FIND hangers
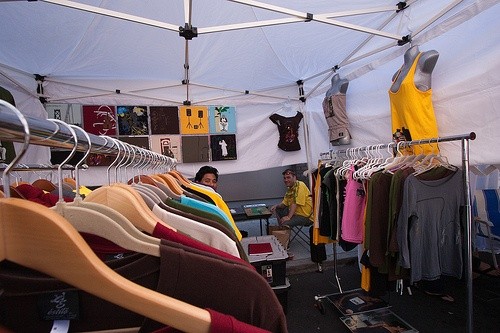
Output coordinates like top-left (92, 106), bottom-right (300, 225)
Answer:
top-left (0, 97), bottom-right (211, 332)
top-left (317, 137), bottom-right (458, 180)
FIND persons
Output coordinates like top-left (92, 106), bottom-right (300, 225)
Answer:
top-left (389, 45), bottom-right (440, 155)
top-left (270, 168), bottom-right (314, 251)
top-left (269, 104), bottom-right (304, 153)
top-left (322, 73), bottom-right (352, 146)
top-left (194, 166), bottom-right (218, 190)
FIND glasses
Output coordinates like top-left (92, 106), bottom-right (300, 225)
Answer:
top-left (282, 172), bottom-right (295, 175)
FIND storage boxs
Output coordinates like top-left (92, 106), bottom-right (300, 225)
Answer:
top-left (271, 277), bottom-right (291, 314)
top-left (241, 235), bottom-right (290, 287)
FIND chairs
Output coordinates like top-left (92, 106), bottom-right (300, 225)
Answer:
top-left (471, 189), bottom-right (500, 270)
top-left (278, 208), bottom-right (313, 251)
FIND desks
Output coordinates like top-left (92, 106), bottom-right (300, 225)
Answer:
top-left (229, 207), bottom-right (272, 236)
